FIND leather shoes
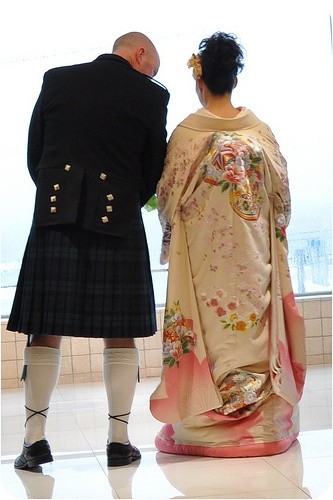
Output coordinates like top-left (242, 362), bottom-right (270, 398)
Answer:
top-left (14, 437), bottom-right (53, 470)
top-left (107, 438), bottom-right (142, 466)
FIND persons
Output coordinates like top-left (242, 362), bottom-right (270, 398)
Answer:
top-left (5, 31), bottom-right (169, 468)
top-left (148, 31), bottom-right (306, 457)
